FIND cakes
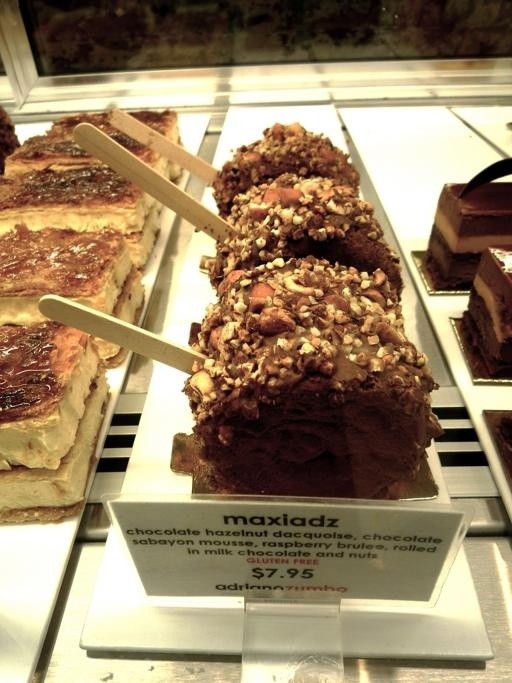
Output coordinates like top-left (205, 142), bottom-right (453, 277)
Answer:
top-left (208, 170), bottom-right (404, 301)
top-left (461, 245), bottom-right (512, 378)
top-left (0, 224), bottom-right (145, 367)
top-left (212, 122), bottom-right (361, 222)
top-left (47, 111), bottom-right (185, 186)
top-left (4, 135), bottom-right (172, 178)
top-left (185, 254), bottom-right (443, 500)
top-left (425, 182), bottom-right (512, 291)
top-left (0, 323), bottom-right (109, 524)
top-left (0, 167), bottom-right (164, 265)
